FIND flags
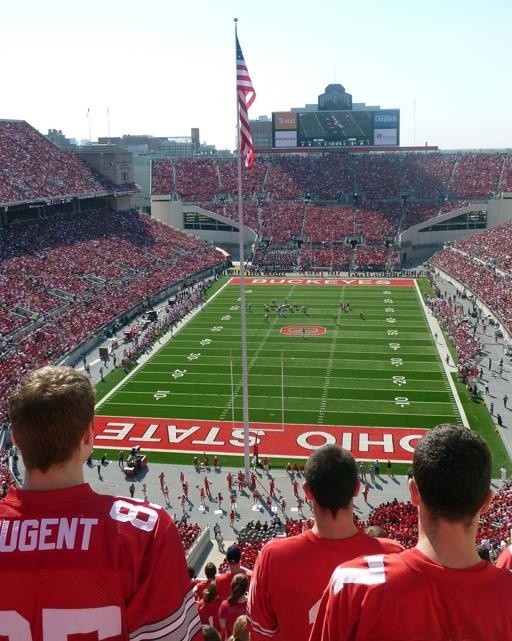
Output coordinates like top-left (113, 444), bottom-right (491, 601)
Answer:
top-left (235, 33), bottom-right (259, 170)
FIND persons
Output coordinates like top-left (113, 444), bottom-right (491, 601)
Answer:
top-left (1, 121), bottom-right (512, 640)
top-left (310, 425), bottom-right (512, 641)
top-left (1, 364), bottom-right (202, 641)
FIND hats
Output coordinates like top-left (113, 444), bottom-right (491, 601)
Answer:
top-left (226, 544), bottom-right (241, 563)
